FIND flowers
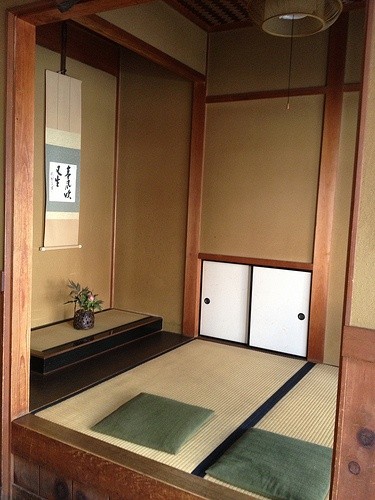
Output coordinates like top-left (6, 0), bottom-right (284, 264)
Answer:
top-left (64, 280), bottom-right (103, 314)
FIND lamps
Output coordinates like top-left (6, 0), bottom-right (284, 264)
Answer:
top-left (247, 0), bottom-right (342, 36)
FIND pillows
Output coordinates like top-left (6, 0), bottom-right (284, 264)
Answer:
top-left (90, 392), bottom-right (215, 455)
top-left (205, 428), bottom-right (333, 500)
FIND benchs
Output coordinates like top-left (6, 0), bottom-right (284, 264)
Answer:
top-left (29, 308), bottom-right (162, 375)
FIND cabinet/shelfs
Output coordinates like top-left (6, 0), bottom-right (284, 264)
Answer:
top-left (199, 258), bottom-right (311, 358)
